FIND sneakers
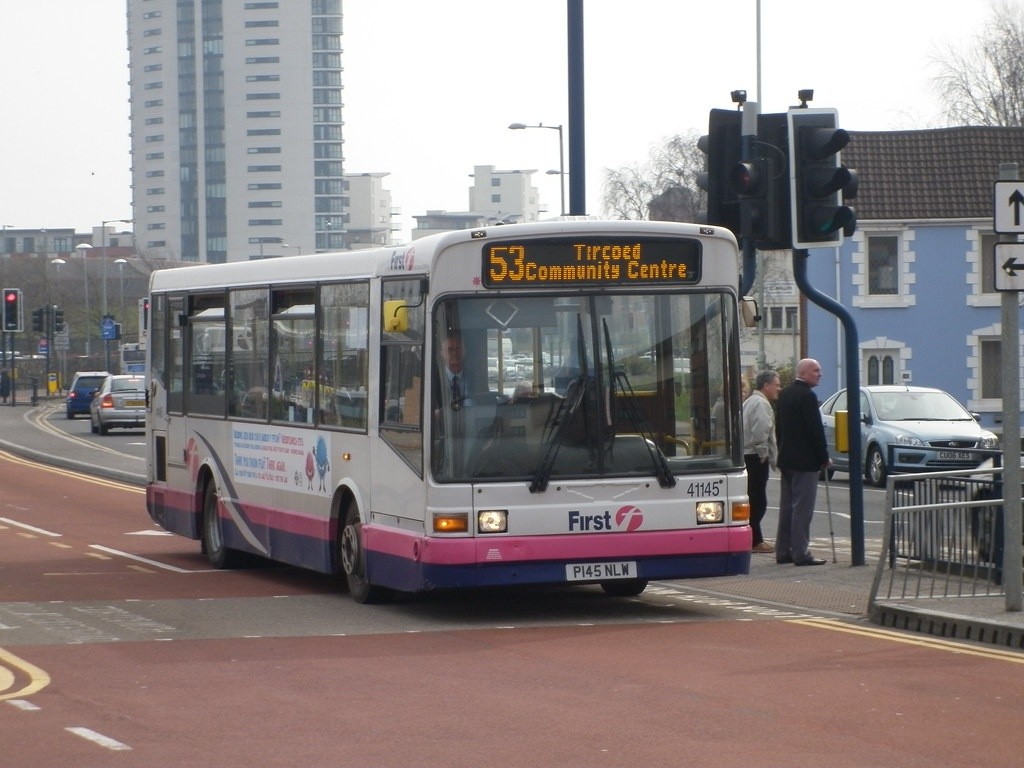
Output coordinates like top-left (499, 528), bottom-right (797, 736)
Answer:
top-left (752, 542), bottom-right (776, 552)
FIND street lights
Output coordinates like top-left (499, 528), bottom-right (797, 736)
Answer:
top-left (75, 219), bottom-right (131, 370)
top-left (509, 122), bottom-right (564, 216)
top-left (50, 259), bottom-right (66, 288)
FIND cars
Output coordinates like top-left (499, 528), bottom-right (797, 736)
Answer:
top-left (965, 446), bottom-right (1024, 565)
top-left (0, 350), bottom-right (47, 360)
top-left (89, 374), bottom-right (146, 435)
top-left (601, 347), bottom-right (691, 380)
top-left (486, 350), bottom-right (564, 382)
top-left (819, 384), bottom-right (999, 488)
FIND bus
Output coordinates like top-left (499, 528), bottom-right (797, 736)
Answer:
top-left (190, 325), bottom-right (280, 403)
top-left (120, 343), bottom-right (145, 374)
top-left (146, 217), bottom-right (762, 604)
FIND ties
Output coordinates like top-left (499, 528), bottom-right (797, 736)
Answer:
top-left (452, 376), bottom-right (460, 401)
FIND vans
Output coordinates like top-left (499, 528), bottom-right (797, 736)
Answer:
top-left (487, 338), bottom-right (512, 356)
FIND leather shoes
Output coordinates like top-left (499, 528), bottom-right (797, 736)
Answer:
top-left (777, 557), bottom-right (793, 564)
top-left (795, 556), bottom-right (826, 566)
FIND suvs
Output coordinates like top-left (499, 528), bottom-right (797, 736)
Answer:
top-left (66, 371), bottom-right (109, 419)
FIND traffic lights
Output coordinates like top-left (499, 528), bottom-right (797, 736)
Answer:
top-left (787, 108), bottom-right (853, 249)
top-left (698, 108), bottom-right (742, 233)
top-left (53, 305), bottom-right (64, 333)
top-left (33, 308), bottom-right (44, 331)
top-left (3, 288), bottom-right (20, 332)
top-left (735, 156), bottom-right (774, 239)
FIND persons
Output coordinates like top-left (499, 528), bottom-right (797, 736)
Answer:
top-left (743, 370), bottom-right (781, 552)
top-left (711, 377), bottom-right (750, 453)
top-left (513, 380), bottom-right (538, 398)
top-left (0, 367), bottom-right (10, 403)
top-left (775, 358), bottom-right (833, 567)
top-left (434, 336), bottom-right (513, 416)
